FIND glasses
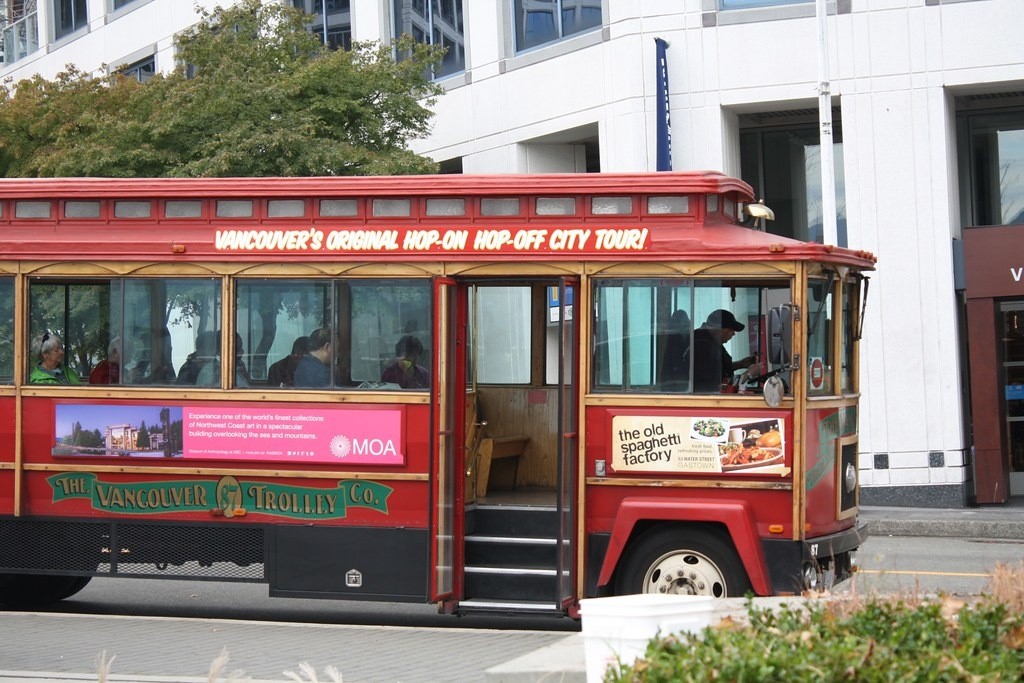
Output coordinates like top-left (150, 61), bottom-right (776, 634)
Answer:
top-left (39, 333), bottom-right (49, 354)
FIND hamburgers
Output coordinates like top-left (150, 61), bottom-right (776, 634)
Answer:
top-left (756, 431), bottom-right (781, 449)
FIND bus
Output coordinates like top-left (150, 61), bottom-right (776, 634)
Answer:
top-left (0, 171), bottom-right (880, 623)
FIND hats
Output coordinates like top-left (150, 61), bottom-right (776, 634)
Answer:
top-left (707, 310), bottom-right (745, 332)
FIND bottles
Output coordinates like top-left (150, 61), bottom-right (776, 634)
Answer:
top-left (769, 423), bottom-right (778, 431)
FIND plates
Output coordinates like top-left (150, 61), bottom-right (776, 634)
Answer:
top-left (719, 447), bottom-right (783, 471)
top-left (691, 418), bottom-right (729, 443)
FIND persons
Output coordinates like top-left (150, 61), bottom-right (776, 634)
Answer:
top-left (29, 332), bottom-right (81, 385)
top-left (266, 336), bottom-right (310, 387)
top-left (293, 328), bottom-right (346, 388)
top-left (175, 330), bottom-right (216, 385)
top-left (684, 308), bottom-right (765, 394)
top-left (197, 329), bottom-right (251, 388)
top-left (382, 335), bottom-right (433, 390)
top-left (89, 336), bottom-right (133, 384)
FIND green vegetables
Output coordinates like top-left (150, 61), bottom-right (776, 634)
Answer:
top-left (695, 420), bottom-right (725, 437)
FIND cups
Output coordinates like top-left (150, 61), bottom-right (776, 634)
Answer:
top-left (729, 428), bottom-right (747, 442)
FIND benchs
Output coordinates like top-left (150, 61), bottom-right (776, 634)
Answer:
top-left (465, 388), bottom-right (529, 498)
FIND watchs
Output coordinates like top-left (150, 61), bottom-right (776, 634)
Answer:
top-left (745, 371), bottom-right (753, 380)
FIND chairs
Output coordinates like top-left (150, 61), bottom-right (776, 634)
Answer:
top-left (662, 310), bottom-right (695, 394)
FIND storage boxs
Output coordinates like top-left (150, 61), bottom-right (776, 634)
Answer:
top-left (577, 595), bottom-right (710, 683)
